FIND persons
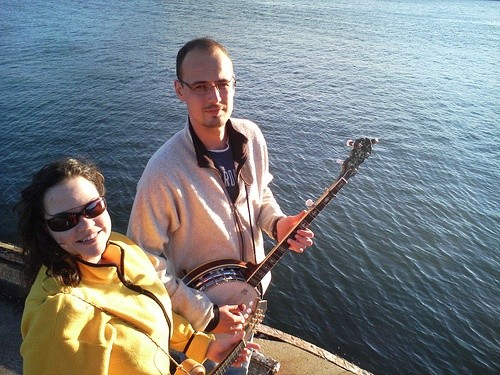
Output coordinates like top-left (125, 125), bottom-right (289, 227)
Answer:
top-left (21, 156), bottom-right (261, 375)
top-left (126, 37), bottom-right (314, 374)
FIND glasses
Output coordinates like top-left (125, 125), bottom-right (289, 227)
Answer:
top-left (45, 196), bottom-right (107, 232)
top-left (181, 76), bottom-right (237, 94)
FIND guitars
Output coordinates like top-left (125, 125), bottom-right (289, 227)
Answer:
top-left (168, 296), bottom-right (270, 375)
